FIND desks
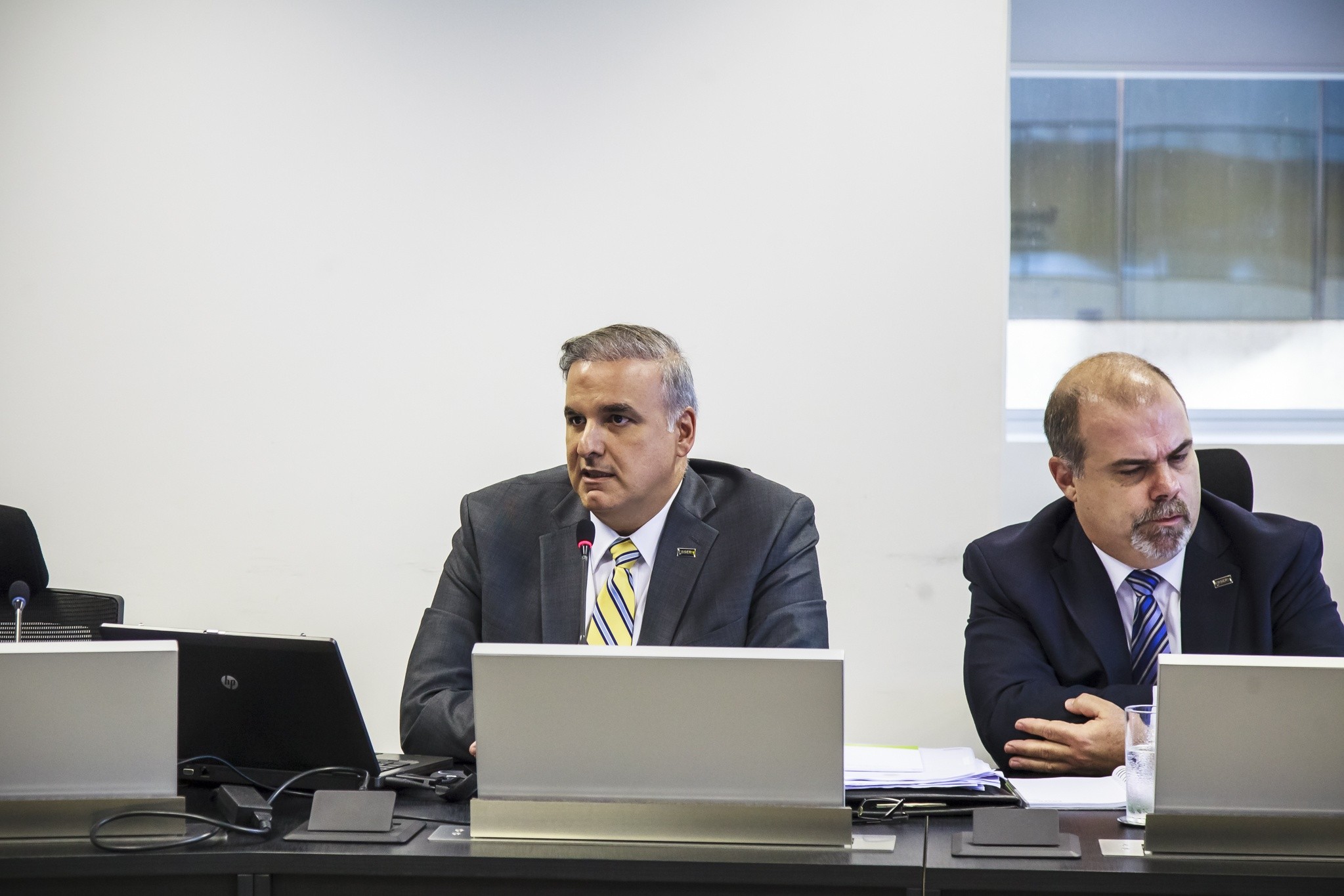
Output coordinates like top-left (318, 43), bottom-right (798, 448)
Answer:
top-left (0, 790), bottom-right (1344, 896)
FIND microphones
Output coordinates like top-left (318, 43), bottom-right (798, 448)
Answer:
top-left (8, 580), bottom-right (30, 643)
top-left (575, 519), bottom-right (595, 645)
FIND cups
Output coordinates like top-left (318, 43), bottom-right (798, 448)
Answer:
top-left (1126, 703), bottom-right (1159, 821)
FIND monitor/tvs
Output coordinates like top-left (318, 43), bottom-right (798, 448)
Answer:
top-left (471, 642), bottom-right (845, 807)
top-left (1152, 653), bottom-right (1344, 814)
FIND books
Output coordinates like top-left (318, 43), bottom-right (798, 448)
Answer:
top-left (844, 743), bottom-right (1139, 816)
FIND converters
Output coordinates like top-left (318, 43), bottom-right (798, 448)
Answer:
top-left (215, 785), bottom-right (274, 827)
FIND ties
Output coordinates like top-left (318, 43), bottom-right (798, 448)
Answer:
top-left (581, 538), bottom-right (643, 645)
top-left (1123, 568), bottom-right (1171, 688)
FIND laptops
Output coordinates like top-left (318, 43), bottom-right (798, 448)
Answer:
top-left (99, 624), bottom-right (453, 790)
top-left (0, 639), bottom-right (178, 797)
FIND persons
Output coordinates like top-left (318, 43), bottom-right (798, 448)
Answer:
top-left (963, 352), bottom-right (1344, 780)
top-left (401, 325), bottom-right (827, 759)
